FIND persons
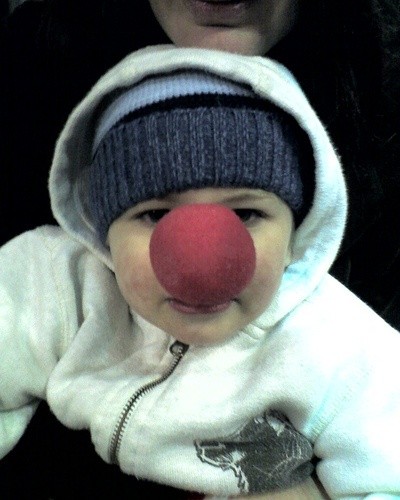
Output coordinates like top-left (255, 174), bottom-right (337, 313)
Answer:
top-left (0, 0), bottom-right (400, 496)
top-left (0, 46), bottom-right (400, 499)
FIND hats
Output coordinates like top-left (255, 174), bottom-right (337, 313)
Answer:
top-left (88, 69), bottom-right (306, 248)
